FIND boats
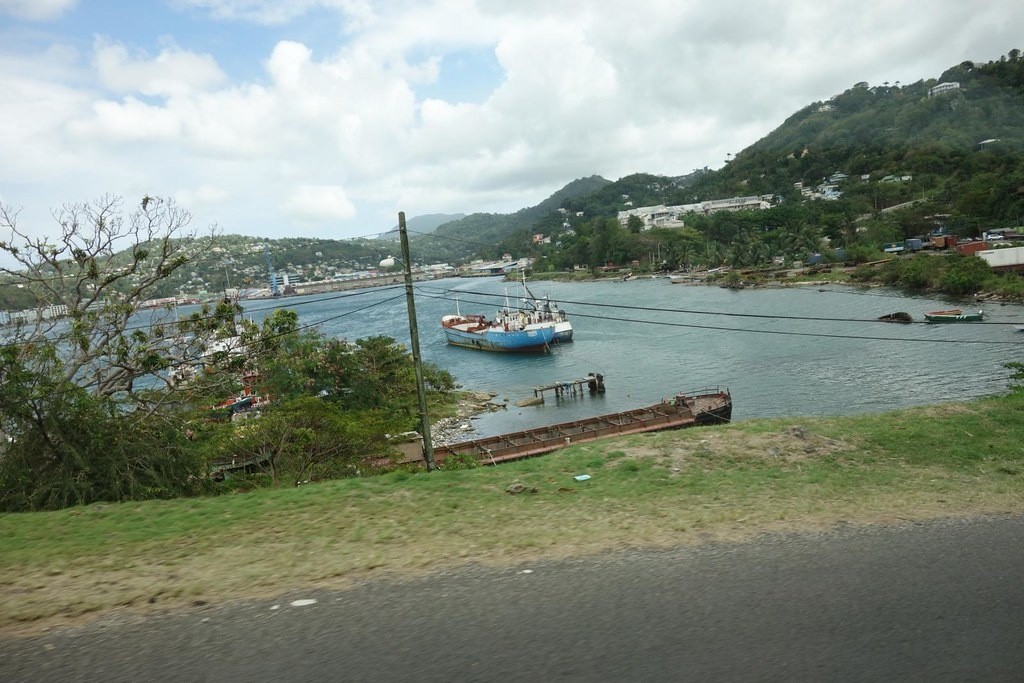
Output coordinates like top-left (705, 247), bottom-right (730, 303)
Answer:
top-left (358, 385), bottom-right (733, 477)
top-left (441, 288), bottom-right (556, 353)
top-left (496, 261), bottom-right (574, 343)
top-left (924, 310), bottom-right (983, 322)
